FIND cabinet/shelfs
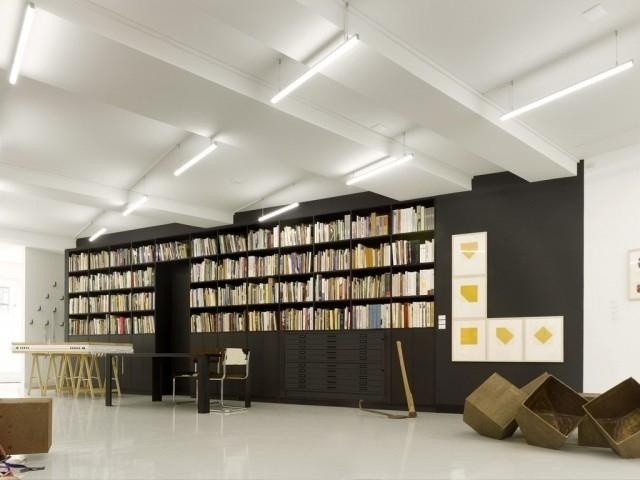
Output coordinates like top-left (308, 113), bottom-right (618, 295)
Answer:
top-left (281, 330), bottom-right (391, 408)
top-left (110, 336), bottom-right (157, 396)
top-left (391, 331), bottom-right (437, 408)
top-left (191, 196), bottom-right (444, 331)
top-left (190, 331), bottom-right (216, 399)
top-left (65, 336), bottom-right (110, 390)
top-left (154, 234), bottom-right (190, 262)
top-left (64, 238), bottom-right (154, 336)
top-left (216, 332), bottom-right (281, 402)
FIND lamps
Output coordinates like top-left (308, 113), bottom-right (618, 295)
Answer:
top-left (174, 138), bottom-right (216, 178)
top-left (257, 182), bottom-right (300, 222)
top-left (88, 218), bottom-right (107, 239)
top-left (500, 30), bottom-right (633, 123)
top-left (346, 131), bottom-right (414, 187)
top-left (123, 179), bottom-right (148, 216)
top-left (271, 2), bottom-right (361, 105)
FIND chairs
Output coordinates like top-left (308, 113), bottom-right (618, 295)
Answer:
top-left (208, 347), bottom-right (253, 409)
top-left (172, 353), bottom-right (222, 411)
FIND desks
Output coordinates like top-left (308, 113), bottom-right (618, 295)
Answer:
top-left (104, 350), bottom-right (222, 414)
top-left (10, 341), bottom-right (135, 399)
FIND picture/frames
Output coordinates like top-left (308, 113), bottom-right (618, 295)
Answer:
top-left (451, 231), bottom-right (489, 276)
top-left (524, 316), bottom-right (565, 363)
top-left (451, 275), bottom-right (488, 319)
top-left (451, 319), bottom-right (485, 363)
top-left (625, 248), bottom-right (640, 302)
top-left (486, 317), bottom-right (524, 362)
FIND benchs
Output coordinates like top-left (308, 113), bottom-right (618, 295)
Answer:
top-left (1, 396), bottom-right (54, 455)
